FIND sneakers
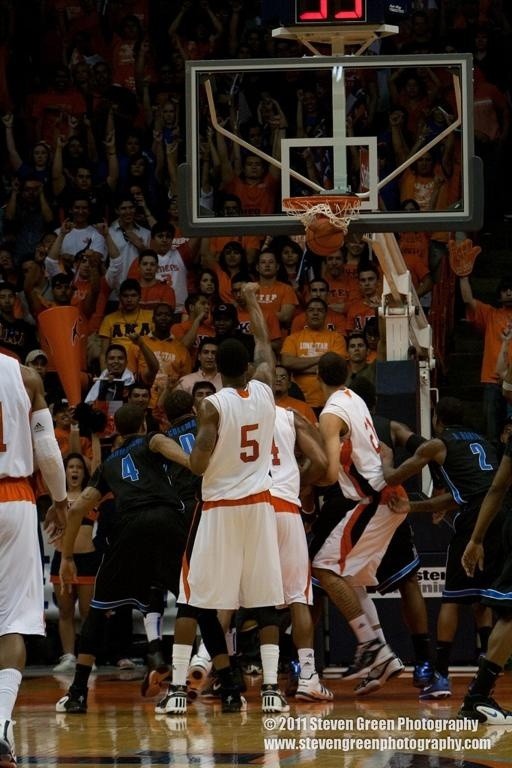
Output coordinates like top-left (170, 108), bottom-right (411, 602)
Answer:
top-left (0, 719), bottom-right (18, 768)
top-left (339, 637), bottom-right (393, 680)
top-left (414, 661), bottom-right (431, 688)
top-left (53, 652), bottom-right (336, 716)
top-left (420, 671), bottom-right (450, 700)
top-left (354, 655), bottom-right (405, 694)
top-left (457, 692), bottom-right (512, 726)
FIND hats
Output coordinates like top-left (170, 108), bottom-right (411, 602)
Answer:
top-left (213, 303), bottom-right (241, 326)
top-left (25, 349), bottom-right (48, 368)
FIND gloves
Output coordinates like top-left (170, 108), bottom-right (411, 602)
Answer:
top-left (450, 238), bottom-right (482, 277)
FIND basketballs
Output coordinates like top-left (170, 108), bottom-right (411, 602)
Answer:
top-left (306, 217), bottom-right (344, 256)
